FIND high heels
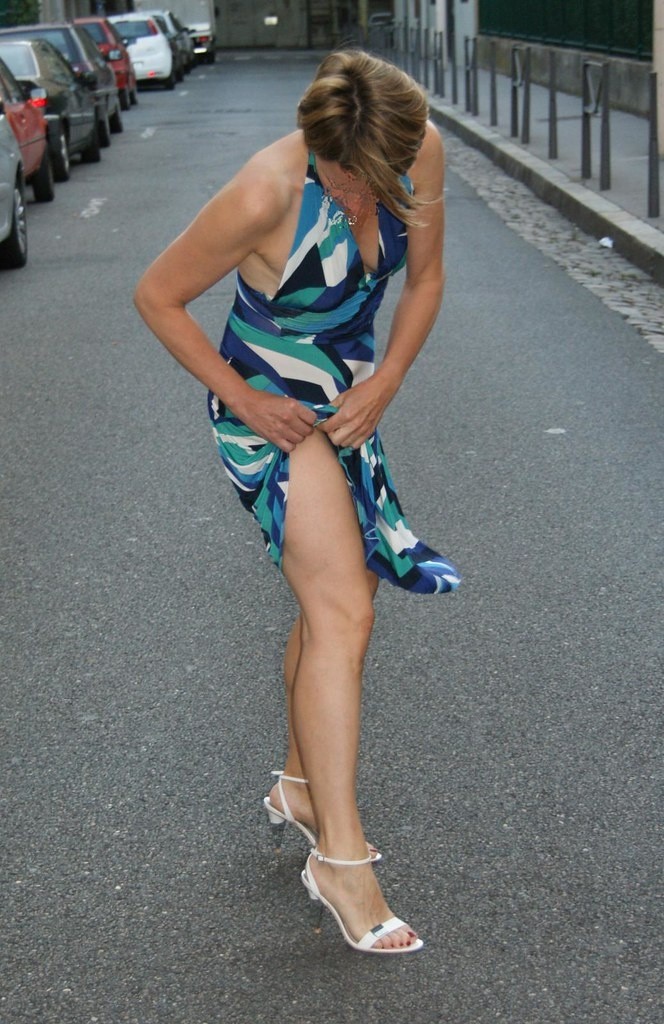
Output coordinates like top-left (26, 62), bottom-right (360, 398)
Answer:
top-left (302, 846), bottom-right (425, 954)
top-left (264, 770), bottom-right (383, 862)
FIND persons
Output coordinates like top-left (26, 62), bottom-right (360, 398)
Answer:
top-left (133, 45), bottom-right (465, 957)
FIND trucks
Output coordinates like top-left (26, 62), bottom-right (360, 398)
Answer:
top-left (136, 0), bottom-right (223, 64)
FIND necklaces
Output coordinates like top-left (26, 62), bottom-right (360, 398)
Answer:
top-left (346, 208), bottom-right (364, 227)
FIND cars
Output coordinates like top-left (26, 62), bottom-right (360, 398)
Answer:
top-left (0, 101), bottom-right (28, 267)
top-left (134, 10), bottom-right (196, 73)
top-left (0, 23), bottom-right (124, 148)
top-left (0, 57), bottom-right (56, 202)
top-left (1, 39), bottom-right (102, 182)
top-left (68, 16), bottom-right (137, 112)
top-left (106, 14), bottom-right (183, 90)
